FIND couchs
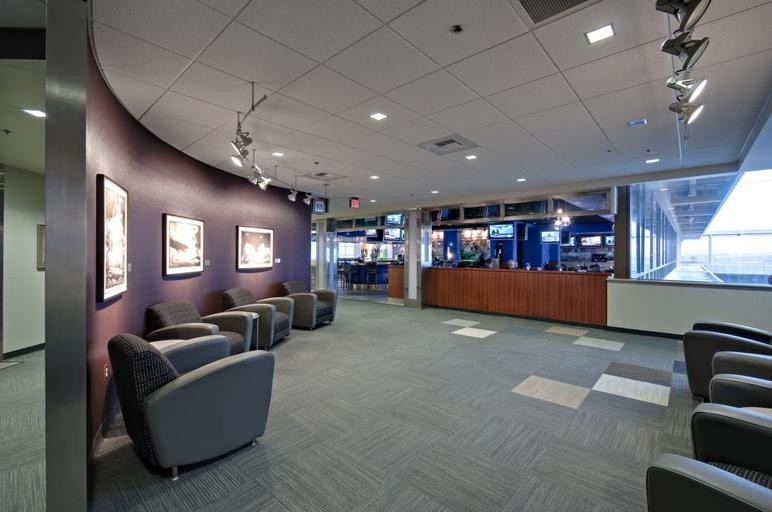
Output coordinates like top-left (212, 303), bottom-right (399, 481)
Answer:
top-left (107, 332), bottom-right (274, 481)
top-left (225, 287), bottom-right (292, 349)
top-left (282, 280), bottom-right (336, 332)
top-left (148, 298), bottom-right (253, 356)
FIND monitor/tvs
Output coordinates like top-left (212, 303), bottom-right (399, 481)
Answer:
top-left (364, 213), bottom-right (615, 249)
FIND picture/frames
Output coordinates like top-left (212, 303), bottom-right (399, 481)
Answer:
top-left (161, 212), bottom-right (204, 278)
top-left (37, 223), bottom-right (47, 271)
top-left (96, 174), bottom-right (129, 305)
top-left (235, 225), bottom-right (274, 273)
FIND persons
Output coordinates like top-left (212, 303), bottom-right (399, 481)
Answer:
top-left (504, 259), bottom-right (515, 270)
top-left (483, 258), bottom-right (494, 269)
top-left (471, 242), bottom-right (478, 251)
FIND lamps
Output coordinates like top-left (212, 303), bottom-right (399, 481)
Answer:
top-left (655, 0), bottom-right (713, 126)
top-left (303, 193), bottom-right (313, 206)
top-left (230, 113), bottom-right (272, 192)
top-left (287, 188), bottom-right (298, 202)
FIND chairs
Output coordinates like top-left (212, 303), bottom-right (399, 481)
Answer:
top-left (645, 319), bottom-right (772, 512)
top-left (312, 258), bottom-right (388, 290)
